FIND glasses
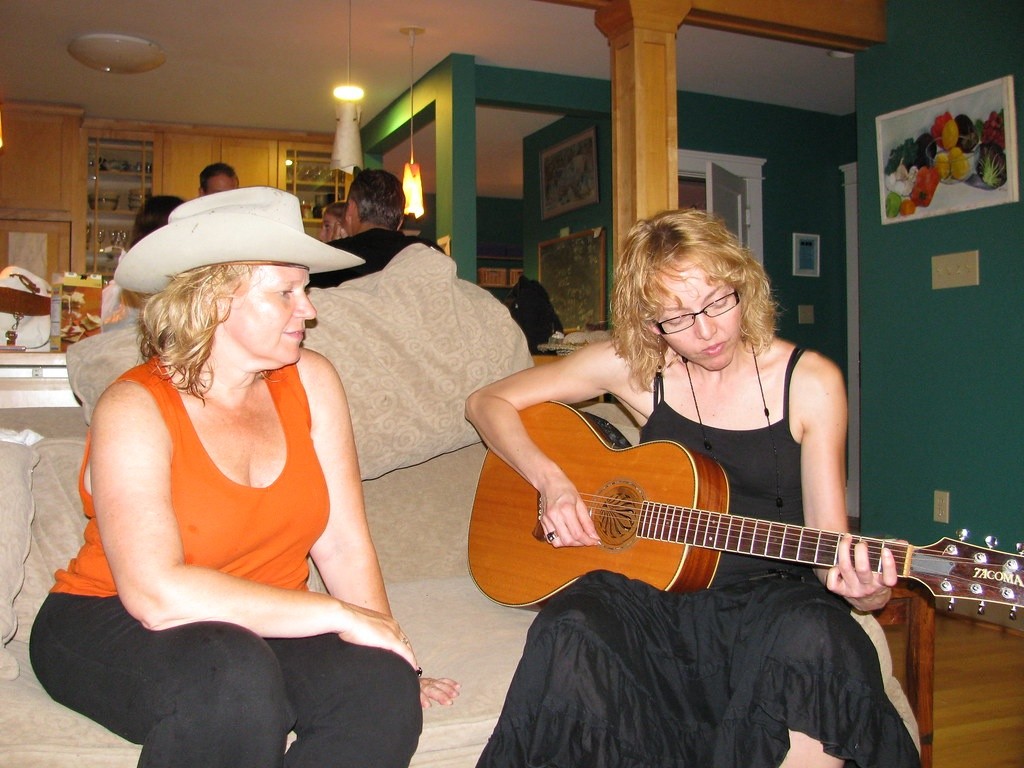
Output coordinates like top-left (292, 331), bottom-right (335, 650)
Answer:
top-left (652, 288), bottom-right (741, 334)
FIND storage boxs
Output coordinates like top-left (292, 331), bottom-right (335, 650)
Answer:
top-left (50, 272), bottom-right (104, 352)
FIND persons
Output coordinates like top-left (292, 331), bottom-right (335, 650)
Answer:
top-left (129, 162), bottom-right (448, 289)
top-left (464, 207), bottom-right (923, 768)
top-left (28, 190), bottom-right (460, 768)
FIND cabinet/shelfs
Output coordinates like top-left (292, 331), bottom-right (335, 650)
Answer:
top-left (476, 254), bottom-right (524, 289)
top-left (0, 101), bottom-right (359, 287)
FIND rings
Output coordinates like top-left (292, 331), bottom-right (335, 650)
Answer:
top-left (547, 530), bottom-right (558, 542)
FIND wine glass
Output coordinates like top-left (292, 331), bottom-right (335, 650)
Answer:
top-left (86, 220), bottom-right (134, 264)
top-left (62, 284), bottom-right (78, 326)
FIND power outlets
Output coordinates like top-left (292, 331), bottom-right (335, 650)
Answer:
top-left (933, 491), bottom-right (949, 524)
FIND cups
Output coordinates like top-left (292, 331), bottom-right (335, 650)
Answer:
top-left (300, 200), bottom-right (311, 216)
top-left (286, 160), bottom-right (346, 184)
top-left (137, 161), bottom-right (150, 174)
top-left (315, 194), bottom-right (327, 204)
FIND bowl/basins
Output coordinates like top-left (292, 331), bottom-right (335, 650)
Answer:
top-left (102, 160), bottom-right (127, 171)
top-left (88, 189), bottom-right (152, 212)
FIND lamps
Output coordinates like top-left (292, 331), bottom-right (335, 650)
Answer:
top-left (401, 26), bottom-right (425, 218)
top-left (67, 34), bottom-right (165, 75)
top-left (330, 0), bottom-right (364, 173)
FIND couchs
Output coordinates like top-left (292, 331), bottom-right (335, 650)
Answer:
top-left (1, 245), bottom-right (938, 767)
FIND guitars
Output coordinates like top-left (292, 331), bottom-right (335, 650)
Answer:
top-left (465, 399), bottom-right (1024, 620)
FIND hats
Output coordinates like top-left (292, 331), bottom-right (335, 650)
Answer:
top-left (115, 186), bottom-right (367, 294)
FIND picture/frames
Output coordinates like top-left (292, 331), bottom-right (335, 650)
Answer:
top-left (876, 75), bottom-right (1019, 226)
top-left (792, 233), bottom-right (820, 279)
top-left (540, 126), bottom-right (598, 220)
top-left (537, 226), bottom-right (607, 332)
